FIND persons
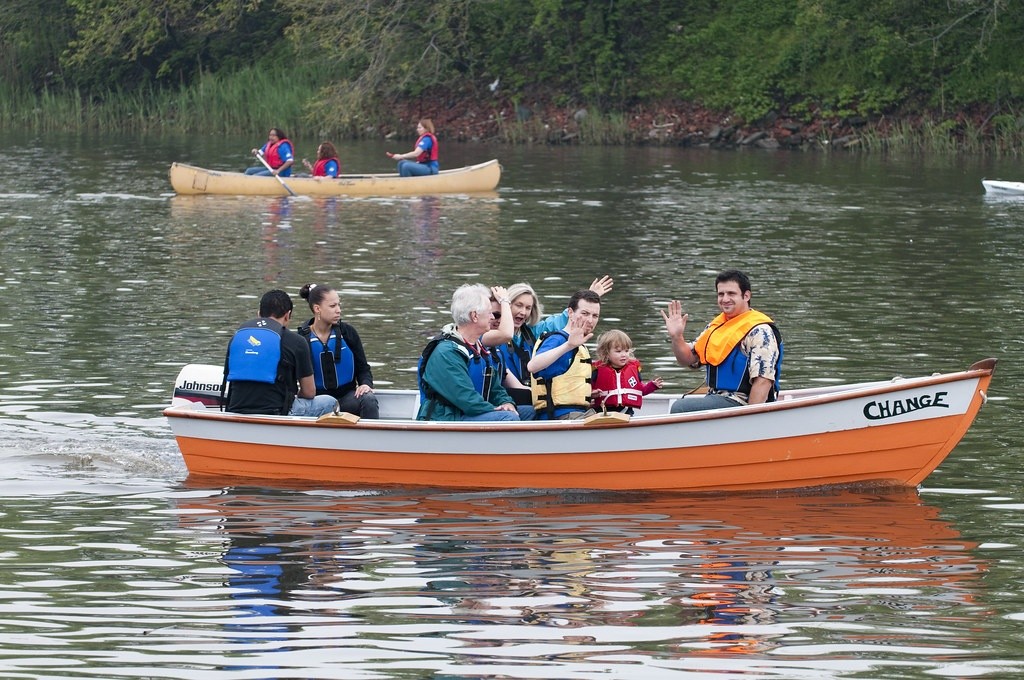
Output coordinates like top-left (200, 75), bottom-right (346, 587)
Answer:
top-left (244, 127), bottom-right (294, 177)
top-left (592, 330), bottom-right (663, 417)
top-left (392, 120), bottom-right (438, 177)
top-left (298, 284), bottom-right (380, 419)
top-left (415, 284), bottom-right (536, 421)
top-left (507, 275), bottom-right (613, 387)
top-left (662, 270), bottom-right (783, 414)
top-left (220, 290), bottom-right (340, 416)
top-left (294, 142), bottom-right (341, 179)
top-left (479, 286), bottom-right (532, 406)
top-left (527, 290), bottom-right (601, 422)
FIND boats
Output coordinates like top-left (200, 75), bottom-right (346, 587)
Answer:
top-left (978, 178), bottom-right (1024, 195)
top-left (169, 158), bottom-right (504, 197)
top-left (161, 354), bottom-right (1000, 490)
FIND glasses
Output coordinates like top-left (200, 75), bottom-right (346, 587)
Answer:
top-left (491, 311), bottom-right (501, 321)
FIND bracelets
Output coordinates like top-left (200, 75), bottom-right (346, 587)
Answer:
top-left (499, 299), bottom-right (511, 304)
